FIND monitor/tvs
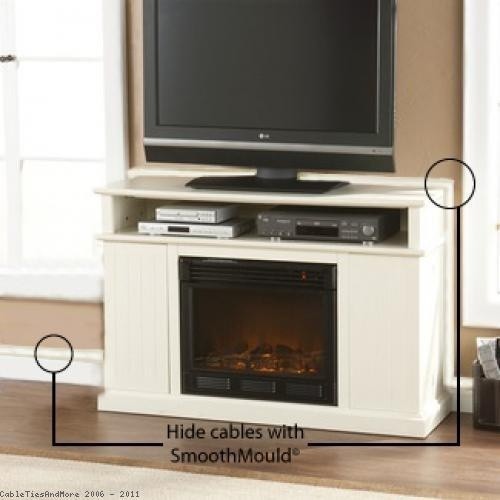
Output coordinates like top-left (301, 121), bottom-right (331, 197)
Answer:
top-left (142, 0), bottom-right (396, 194)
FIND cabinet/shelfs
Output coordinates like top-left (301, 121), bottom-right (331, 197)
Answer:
top-left (95, 165), bottom-right (455, 441)
